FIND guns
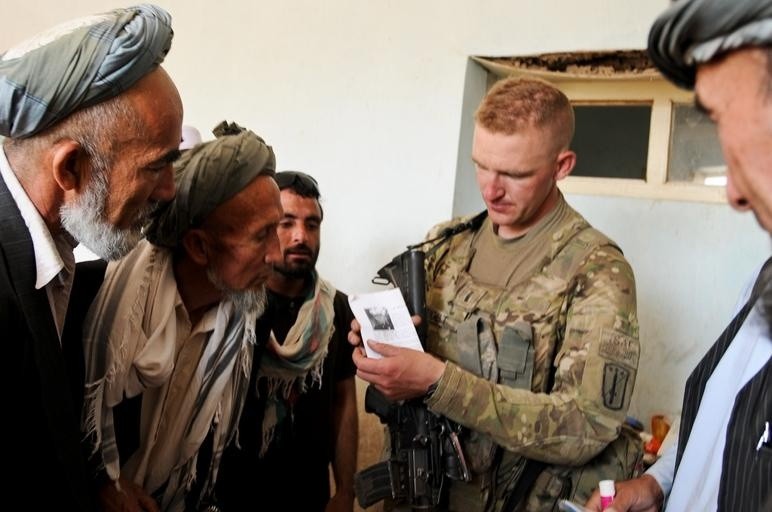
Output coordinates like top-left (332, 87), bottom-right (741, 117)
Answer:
top-left (355, 249), bottom-right (473, 511)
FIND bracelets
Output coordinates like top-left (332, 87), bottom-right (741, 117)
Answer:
top-left (425, 374), bottom-right (443, 399)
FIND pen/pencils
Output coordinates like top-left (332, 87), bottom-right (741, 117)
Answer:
top-left (626, 417), bottom-right (643, 432)
top-left (756, 422), bottom-right (770, 460)
top-left (558, 480), bottom-right (618, 511)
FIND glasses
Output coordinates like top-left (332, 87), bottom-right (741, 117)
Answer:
top-left (274, 171), bottom-right (319, 195)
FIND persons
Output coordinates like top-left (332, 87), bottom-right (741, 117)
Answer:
top-left (346, 77), bottom-right (640, 511)
top-left (73, 119), bottom-right (284, 512)
top-left (581, 0), bottom-right (772, 512)
top-left (0, 2), bottom-right (183, 511)
top-left (182, 171), bottom-right (358, 512)
